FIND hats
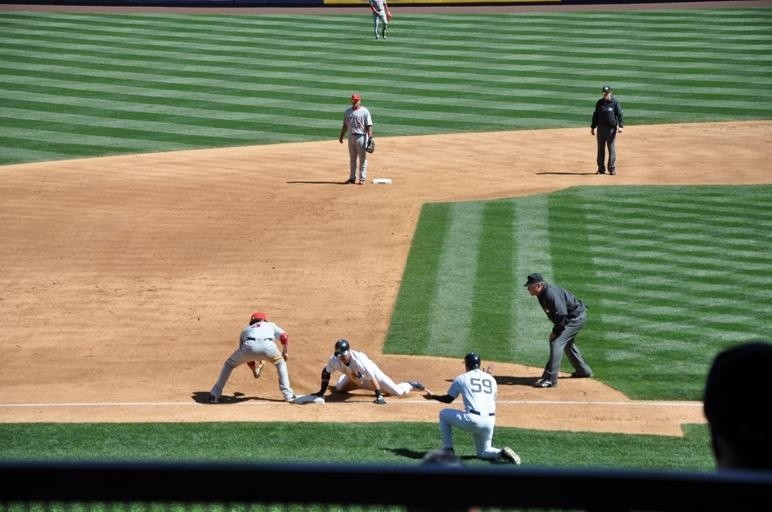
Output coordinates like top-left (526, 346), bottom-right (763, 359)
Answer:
top-left (351, 93), bottom-right (361, 100)
top-left (602, 86), bottom-right (610, 93)
top-left (523, 273), bottom-right (543, 286)
top-left (250, 312), bottom-right (267, 322)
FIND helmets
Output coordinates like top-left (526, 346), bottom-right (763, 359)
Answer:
top-left (334, 339), bottom-right (350, 358)
top-left (462, 353), bottom-right (480, 369)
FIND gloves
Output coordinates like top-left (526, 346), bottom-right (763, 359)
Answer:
top-left (374, 395), bottom-right (386, 405)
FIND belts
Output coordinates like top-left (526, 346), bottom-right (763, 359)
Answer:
top-left (247, 337), bottom-right (272, 341)
top-left (469, 409), bottom-right (495, 416)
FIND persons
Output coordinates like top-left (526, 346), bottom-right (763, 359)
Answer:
top-left (339, 94), bottom-right (374, 185)
top-left (309, 341), bottom-right (426, 404)
top-left (208, 312), bottom-right (304, 403)
top-left (591, 85), bottom-right (623, 174)
top-left (420, 354), bottom-right (520, 465)
top-left (403, 452), bottom-right (480, 512)
top-left (523, 274), bottom-right (591, 387)
top-left (370, 0), bottom-right (390, 40)
top-left (704, 344), bottom-right (770, 474)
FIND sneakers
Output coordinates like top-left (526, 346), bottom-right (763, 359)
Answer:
top-left (500, 447), bottom-right (521, 465)
top-left (533, 378), bottom-right (553, 387)
top-left (206, 396), bottom-right (220, 403)
top-left (345, 178), bottom-right (356, 184)
top-left (284, 394), bottom-right (296, 402)
top-left (359, 180), bottom-right (366, 185)
top-left (408, 381), bottom-right (427, 391)
